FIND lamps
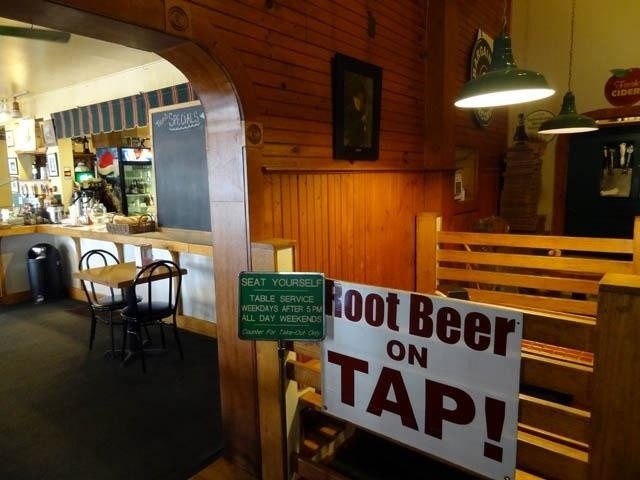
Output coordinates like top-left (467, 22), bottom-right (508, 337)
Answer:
top-left (450, 3), bottom-right (555, 114)
top-left (536, 1), bottom-right (599, 141)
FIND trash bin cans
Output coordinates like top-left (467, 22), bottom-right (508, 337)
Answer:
top-left (25, 243), bottom-right (70, 307)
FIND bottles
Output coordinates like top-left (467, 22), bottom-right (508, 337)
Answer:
top-left (129, 179), bottom-right (152, 194)
top-left (513, 112), bottom-right (529, 151)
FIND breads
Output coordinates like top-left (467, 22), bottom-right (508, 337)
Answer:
top-left (112, 214), bottom-right (148, 225)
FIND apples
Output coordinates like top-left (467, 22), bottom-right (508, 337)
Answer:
top-left (604, 67), bottom-right (640, 106)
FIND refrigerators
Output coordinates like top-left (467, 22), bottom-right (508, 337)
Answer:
top-left (94, 146), bottom-right (157, 226)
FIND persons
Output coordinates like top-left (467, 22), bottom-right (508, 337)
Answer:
top-left (344, 78), bottom-right (374, 150)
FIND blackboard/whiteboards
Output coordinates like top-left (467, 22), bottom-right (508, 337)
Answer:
top-left (238, 269), bottom-right (326, 342)
top-left (147, 102), bottom-right (212, 238)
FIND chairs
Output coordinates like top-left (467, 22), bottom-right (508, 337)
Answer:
top-left (71, 249), bottom-right (189, 374)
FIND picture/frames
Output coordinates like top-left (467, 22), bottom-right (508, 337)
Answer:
top-left (331, 50), bottom-right (384, 162)
top-left (44, 152), bottom-right (60, 178)
top-left (38, 118), bottom-right (58, 147)
